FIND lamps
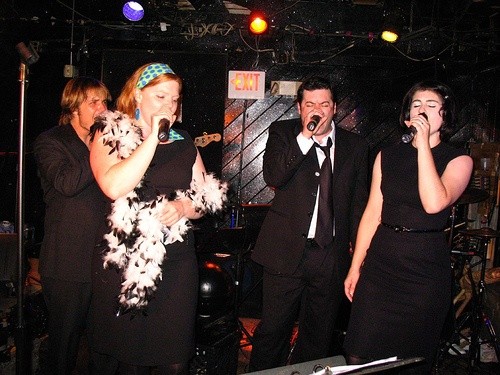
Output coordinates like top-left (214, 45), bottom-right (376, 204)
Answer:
top-left (16, 42), bottom-right (33, 61)
top-left (381, 13), bottom-right (401, 43)
top-left (122, 0), bottom-right (147, 22)
top-left (249, 10), bottom-right (267, 35)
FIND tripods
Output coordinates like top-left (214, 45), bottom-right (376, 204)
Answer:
top-left (435, 237), bottom-right (500, 372)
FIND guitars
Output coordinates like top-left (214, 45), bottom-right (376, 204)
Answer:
top-left (194, 132), bottom-right (222, 148)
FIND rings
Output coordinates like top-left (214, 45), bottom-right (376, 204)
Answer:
top-left (420, 123), bottom-right (423, 126)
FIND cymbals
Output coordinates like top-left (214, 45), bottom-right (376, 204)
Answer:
top-left (455, 228), bottom-right (500, 239)
top-left (453, 187), bottom-right (490, 205)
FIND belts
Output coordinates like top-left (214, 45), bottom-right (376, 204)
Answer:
top-left (381, 221), bottom-right (443, 233)
top-left (305, 237), bottom-right (335, 247)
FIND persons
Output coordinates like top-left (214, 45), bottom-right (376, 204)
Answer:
top-left (344, 80), bottom-right (474, 375)
top-left (89, 63), bottom-right (208, 375)
top-left (33, 74), bottom-right (112, 375)
top-left (246, 75), bottom-right (371, 372)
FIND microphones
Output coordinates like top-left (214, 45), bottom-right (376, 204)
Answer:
top-left (158, 118), bottom-right (170, 142)
top-left (402, 113), bottom-right (428, 143)
top-left (307, 114), bottom-right (321, 131)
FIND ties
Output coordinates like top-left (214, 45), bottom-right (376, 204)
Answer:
top-left (310, 136), bottom-right (335, 249)
